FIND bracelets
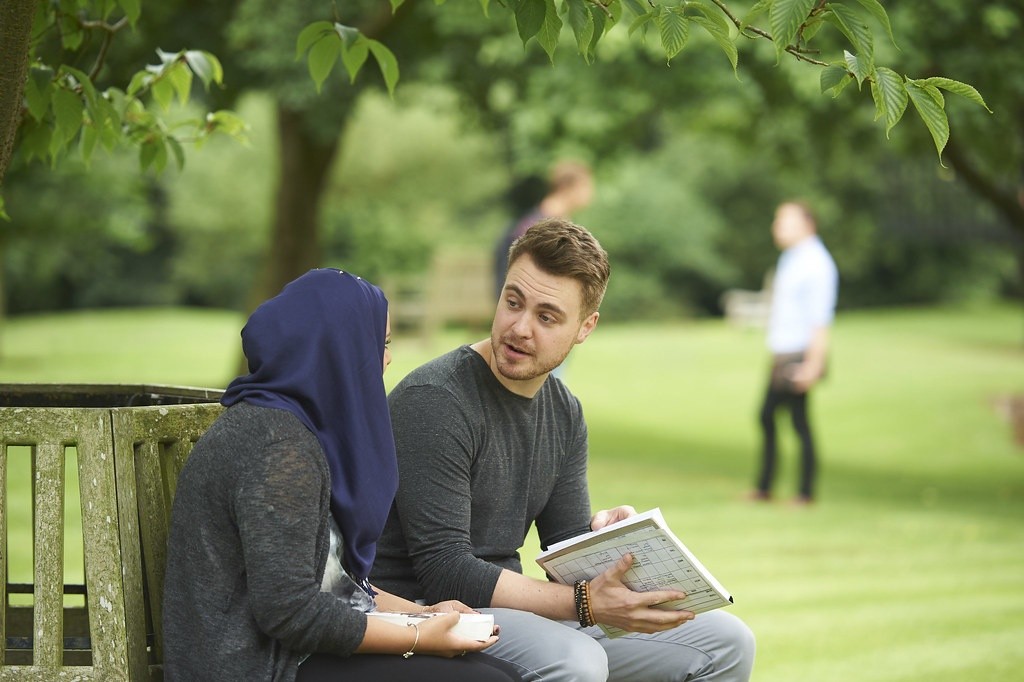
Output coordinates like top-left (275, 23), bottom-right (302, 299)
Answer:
top-left (401, 621), bottom-right (420, 658)
top-left (574, 579), bottom-right (597, 628)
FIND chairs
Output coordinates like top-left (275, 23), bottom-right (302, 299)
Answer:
top-left (1, 383), bottom-right (235, 682)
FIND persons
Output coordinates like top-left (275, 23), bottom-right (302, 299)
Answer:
top-left (160, 267), bottom-right (522, 682)
top-left (493, 162), bottom-right (594, 304)
top-left (369, 216), bottom-right (757, 682)
top-left (722, 198), bottom-right (840, 505)
